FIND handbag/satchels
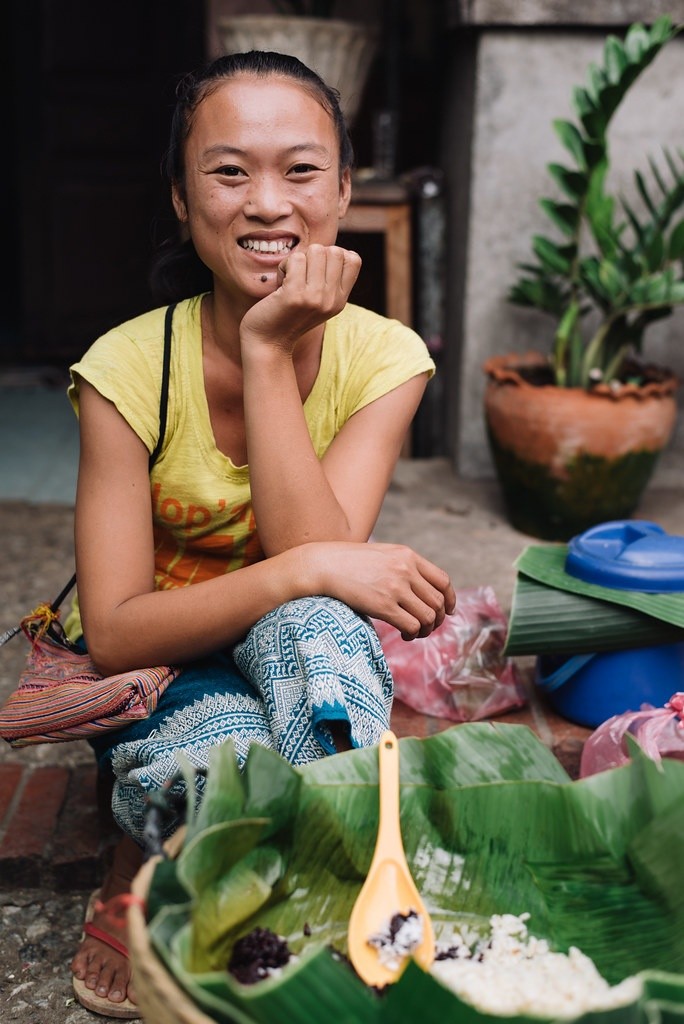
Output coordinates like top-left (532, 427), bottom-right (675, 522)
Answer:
top-left (0, 623), bottom-right (186, 749)
top-left (367, 584), bottom-right (527, 722)
top-left (579, 691), bottom-right (684, 780)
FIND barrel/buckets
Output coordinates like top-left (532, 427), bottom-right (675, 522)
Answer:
top-left (529, 518), bottom-right (684, 729)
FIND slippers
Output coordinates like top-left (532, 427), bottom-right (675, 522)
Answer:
top-left (73, 887), bottom-right (143, 1018)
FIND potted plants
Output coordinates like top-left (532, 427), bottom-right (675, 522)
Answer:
top-left (480, 14), bottom-right (684, 543)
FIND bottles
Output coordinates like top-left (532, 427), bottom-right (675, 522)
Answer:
top-left (372, 110), bottom-right (399, 180)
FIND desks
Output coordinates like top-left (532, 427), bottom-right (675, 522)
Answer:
top-left (338, 182), bottom-right (410, 458)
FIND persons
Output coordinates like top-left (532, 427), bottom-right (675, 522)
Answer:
top-left (65, 52), bottom-right (459, 1019)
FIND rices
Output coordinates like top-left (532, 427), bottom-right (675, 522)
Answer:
top-left (261, 910), bottom-right (616, 1014)
